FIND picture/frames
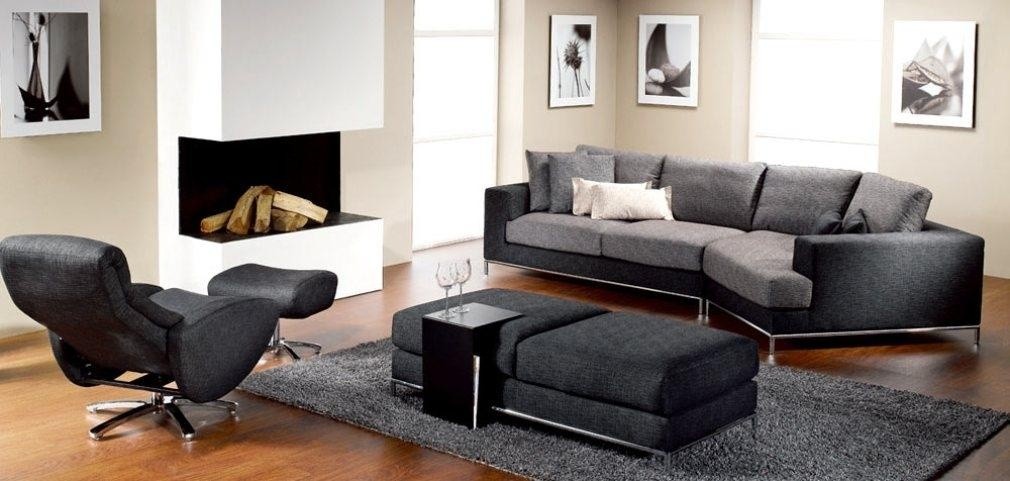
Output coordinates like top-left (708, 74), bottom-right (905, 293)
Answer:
top-left (637, 13), bottom-right (700, 109)
top-left (0, 0), bottom-right (103, 141)
top-left (889, 19), bottom-right (979, 128)
top-left (548, 14), bottom-right (598, 109)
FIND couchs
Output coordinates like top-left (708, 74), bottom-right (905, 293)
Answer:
top-left (482, 144), bottom-right (768, 324)
top-left (390, 287), bottom-right (612, 424)
top-left (207, 263), bottom-right (338, 362)
top-left (485, 309), bottom-right (760, 461)
top-left (0, 233), bottom-right (283, 440)
top-left (703, 162), bottom-right (988, 357)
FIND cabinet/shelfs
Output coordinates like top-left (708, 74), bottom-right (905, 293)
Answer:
top-left (178, 212), bottom-right (384, 302)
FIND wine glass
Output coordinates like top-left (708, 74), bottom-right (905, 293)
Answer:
top-left (450, 258), bottom-right (471, 313)
top-left (437, 261), bottom-right (457, 318)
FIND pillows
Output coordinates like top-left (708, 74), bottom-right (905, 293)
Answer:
top-left (524, 149), bottom-right (590, 213)
top-left (545, 152), bottom-right (617, 215)
top-left (841, 209), bottom-right (867, 235)
top-left (571, 176), bottom-right (653, 217)
top-left (588, 185), bottom-right (675, 222)
top-left (812, 208), bottom-right (841, 236)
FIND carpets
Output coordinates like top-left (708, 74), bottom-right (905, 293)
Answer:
top-left (236, 332), bottom-right (1010, 481)
